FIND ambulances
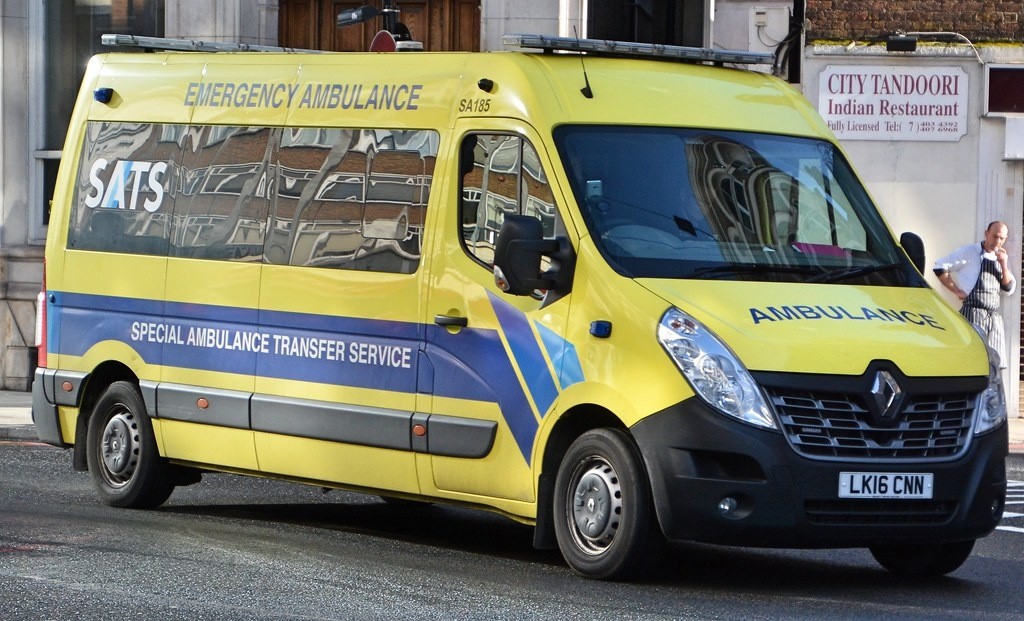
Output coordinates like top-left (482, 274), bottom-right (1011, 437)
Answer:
top-left (27, 35), bottom-right (1012, 581)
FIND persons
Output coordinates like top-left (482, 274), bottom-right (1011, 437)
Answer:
top-left (933, 221), bottom-right (1016, 377)
